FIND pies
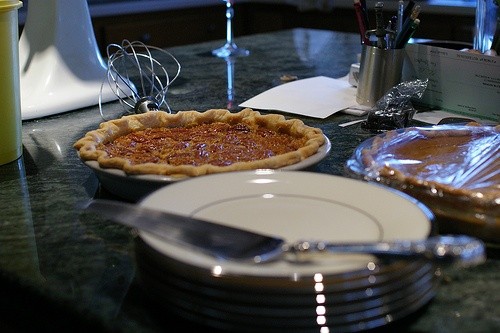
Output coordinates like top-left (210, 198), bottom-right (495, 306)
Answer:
top-left (359, 122), bottom-right (500, 230)
top-left (72, 109), bottom-right (332, 194)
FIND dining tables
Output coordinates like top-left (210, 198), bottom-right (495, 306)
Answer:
top-left (0, 28), bottom-right (500, 333)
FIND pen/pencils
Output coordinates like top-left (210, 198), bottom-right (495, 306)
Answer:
top-left (354, 0), bottom-right (422, 49)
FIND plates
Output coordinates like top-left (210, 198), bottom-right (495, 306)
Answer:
top-left (77, 134), bottom-right (331, 206)
top-left (356, 124), bottom-right (500, 246)
top-left (134, 168), bottom-right (438, 333)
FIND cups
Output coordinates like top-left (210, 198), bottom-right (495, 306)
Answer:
top-left (0, 0), bottom-right (23, 166)
top-left (357, 44), bottom-right (405, 106)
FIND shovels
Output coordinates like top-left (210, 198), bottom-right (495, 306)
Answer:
top-left (76, 197), bottom-right (486, 267)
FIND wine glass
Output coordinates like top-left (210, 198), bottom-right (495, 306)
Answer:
top-left (211, 0), bottom-right (250, 57)
top-left (217, 55), bottom-right (247, 107)
